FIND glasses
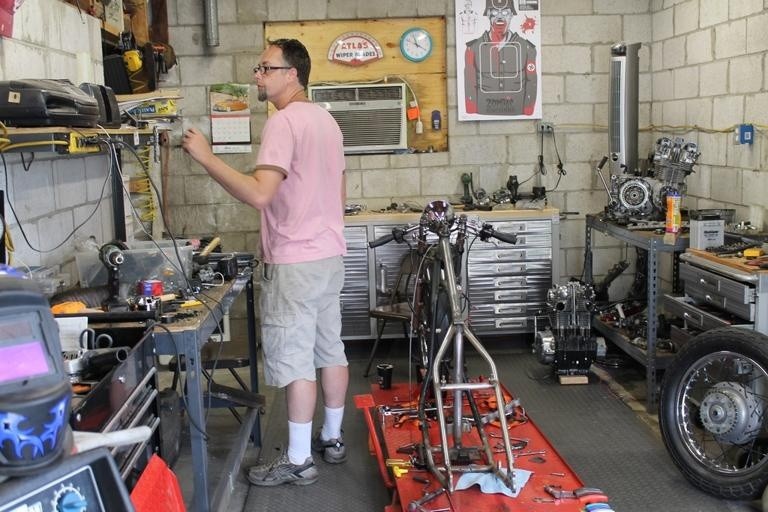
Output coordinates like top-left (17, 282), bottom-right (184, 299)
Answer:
top-left (253, 63), bottom-right (294, 75)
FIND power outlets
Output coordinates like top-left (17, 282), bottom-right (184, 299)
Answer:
top-left (536, 121), bottom-right (553, 133)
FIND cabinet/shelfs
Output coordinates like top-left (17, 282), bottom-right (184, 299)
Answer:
top-left (665, 243), bottom-right (766, 355)
top-left (335, 204), bottom-right (562, 345)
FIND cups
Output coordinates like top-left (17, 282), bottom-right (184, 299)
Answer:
top-left (376, 363), bottom-right (394, 390)
top-left (138, 278), bottom-right (164, 296)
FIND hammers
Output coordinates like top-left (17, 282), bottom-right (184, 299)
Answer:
top-left (193, 237), bottom-right (221, 265)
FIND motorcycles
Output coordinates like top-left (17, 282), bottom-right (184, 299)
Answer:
top-left (366, 194), bottom-right (521, 501)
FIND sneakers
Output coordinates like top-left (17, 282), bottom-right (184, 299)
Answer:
top-left (311, 424), bottom-right (350, 465)
top-left (244, 447), bottom-right (319, 487)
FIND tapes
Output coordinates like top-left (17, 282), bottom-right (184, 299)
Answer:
top-left (161, 315), bottom-right (174, 323)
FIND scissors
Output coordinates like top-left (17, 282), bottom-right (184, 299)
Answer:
top-left (79, 328), bottom-right (113, 348)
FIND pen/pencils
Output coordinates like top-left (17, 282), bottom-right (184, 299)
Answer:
top-left (180, 301), bottom-right (209, 308)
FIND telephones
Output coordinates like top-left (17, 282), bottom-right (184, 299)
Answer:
top-left (0, 78), bottom-right (102, 126)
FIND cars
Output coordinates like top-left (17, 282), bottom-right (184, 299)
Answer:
top-left (214, 99), bottom-right (247, 112)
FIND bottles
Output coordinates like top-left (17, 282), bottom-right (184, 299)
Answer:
top-left (663, 189), bottom-right (683, 235)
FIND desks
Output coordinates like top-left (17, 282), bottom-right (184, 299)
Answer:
top-left (36, 241), bottom-right (264, 511)
top-left (583, 214), bottom-right (740, 415)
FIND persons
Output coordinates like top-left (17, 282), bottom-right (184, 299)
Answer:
top-left (180, 38), bottom-right (349, 487)
top-left (465, 0), bottom-right (538, 116)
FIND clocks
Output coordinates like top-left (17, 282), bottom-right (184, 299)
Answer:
top-left (400, 27), bottom-right (431, 62)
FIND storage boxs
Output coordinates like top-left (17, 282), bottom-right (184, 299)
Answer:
top-left (114, 236), bottom-right (196, 299)
top-left (70, 243), bottom-right (111, 289)
